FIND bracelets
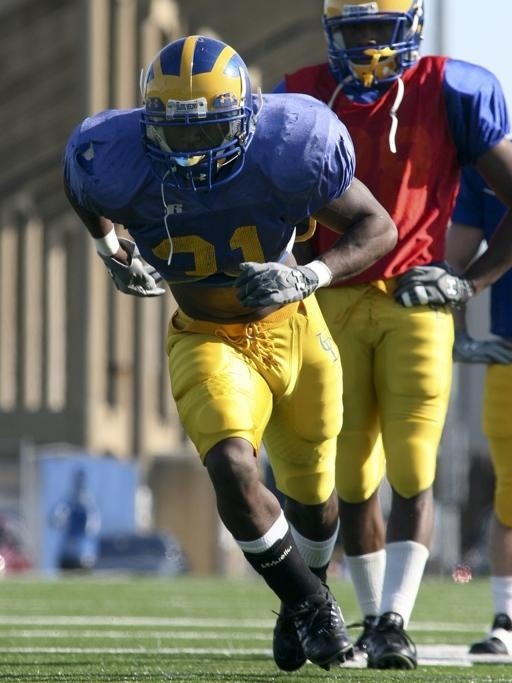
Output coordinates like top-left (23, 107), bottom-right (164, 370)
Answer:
top-left (93, 223), bottom-right (120, 257)
top-left (303, 260), bottom-right (332, 290)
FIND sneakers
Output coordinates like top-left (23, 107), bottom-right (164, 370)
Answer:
top-left (269, 567), bottom-right (353, 673)
top-left (465, 611), bottom-right (512, 665)
top-left (355, 611), bottom-right (421, 671)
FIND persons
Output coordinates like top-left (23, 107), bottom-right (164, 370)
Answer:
top-left (267, 0), bottom-right (512, 671)
top-left (62, 34), bottom-right (399, 672)
top-left (48, 462), bottom-right (103, 570)
top-left (445, 132), bottom-right (510, 667)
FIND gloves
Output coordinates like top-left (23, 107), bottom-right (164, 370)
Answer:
top-left (390, 262), bottom-right (477, 307)
top-left (97, 236), bottom-right (169, 298)
top-left (451, 305), bottom-right (512, 366)
top-left (235, 256), bottom-right (332, 312)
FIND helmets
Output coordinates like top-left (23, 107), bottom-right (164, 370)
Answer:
top-left (323, 0), bottom-right (426, 88)
top-left (139, 34), bottom-right (261, 193)
